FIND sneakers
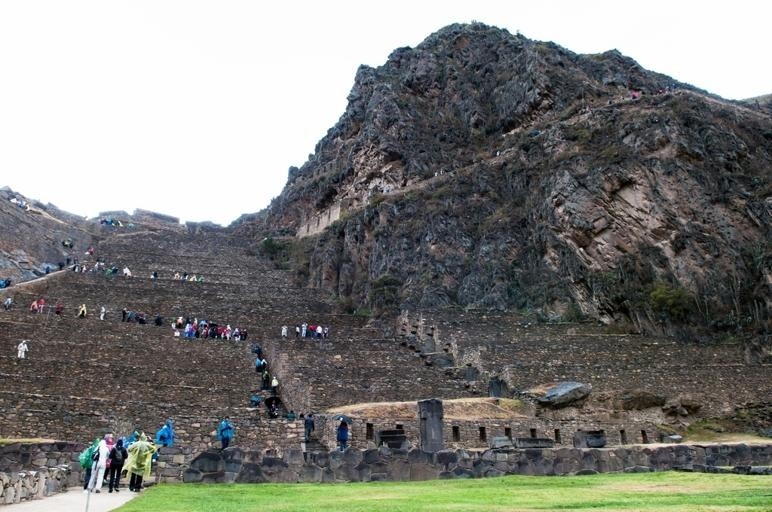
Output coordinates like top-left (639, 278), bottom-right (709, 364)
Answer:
top-left (95, 489), bottom-right (120, 492)
top-left (130, 486), bottom-right (144, 492)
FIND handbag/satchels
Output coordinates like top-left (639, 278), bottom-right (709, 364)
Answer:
top-left (92, 448), bottom-right (100, 461)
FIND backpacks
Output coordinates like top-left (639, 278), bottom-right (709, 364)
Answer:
top-left (114, 447), bottom-right (123, 461)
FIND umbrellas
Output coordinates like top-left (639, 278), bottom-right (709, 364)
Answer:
top-left (333, 415), bottom-right (352, 424)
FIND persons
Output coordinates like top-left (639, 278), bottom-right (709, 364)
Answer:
top-left (25, 298), bottom-right (164, 327)
top-left (338, 421), bottom-right (347, 450)
top-left (46, 254), bottom-right (131, 278)
top-left (153, 419), bottom-right (177, 464)
top-left (170, 316), bottom-right (281, 419)
top-left (295, 320), bottom-right (330, 339)
top-left (79, 428), bottom-right (156, 493)
top-left (174, 269), bottom-right (205, 284)
top-left (287, 409), bottom-right (315, 443)
top-left (99, 219), bottom-right (135, 230)
top-left (4, 296), bottom-right (12, 310)
top-left (218, 416), bottom-right (235, 446)
top-left (16, 339), bottom-right (28, 362)
